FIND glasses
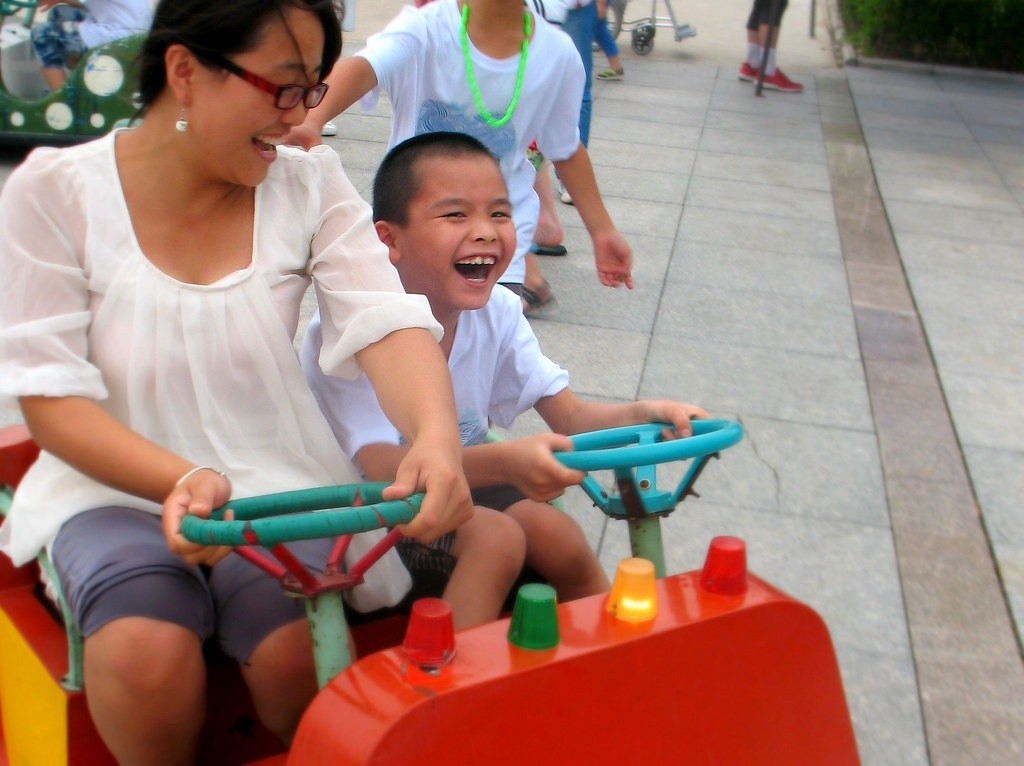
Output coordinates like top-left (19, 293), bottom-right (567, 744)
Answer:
top-left (195, 49), bottom-right (329, 111)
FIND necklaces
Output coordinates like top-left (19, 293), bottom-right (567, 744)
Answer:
top-left (460, 3), bottom-right (532, 132)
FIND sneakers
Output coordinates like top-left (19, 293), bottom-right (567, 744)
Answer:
top-left (739, 62), bottom-right (758, 80)
top-left (755, 67), bottom-right (802, 92)
top-left (596, 67), bottom-right (627, 82)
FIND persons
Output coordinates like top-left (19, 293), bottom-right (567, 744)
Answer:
top-left (278, 0), bottom-right (635, 304)
top-left (2, 0), bottom-right (476, 766)
top-left (414, 0), bottom-right (625, 316)
top-left (293, 131), bottom-right (714, 634)
top-left (30, 0), bottom-right (158, 96)
top-left (737, 0), bottom-right (805, 92)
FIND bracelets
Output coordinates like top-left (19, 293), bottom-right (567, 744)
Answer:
top-left (174, 466), bottom-right (226, 488)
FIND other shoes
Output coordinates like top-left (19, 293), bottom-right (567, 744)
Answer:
top-left (556, 181), bottom-right (571, 203)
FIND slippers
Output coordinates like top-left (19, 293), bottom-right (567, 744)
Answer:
top-left (530, 237), bottom-right (571, 258)
top-left (523, 283), bottom-right (557, 317)
